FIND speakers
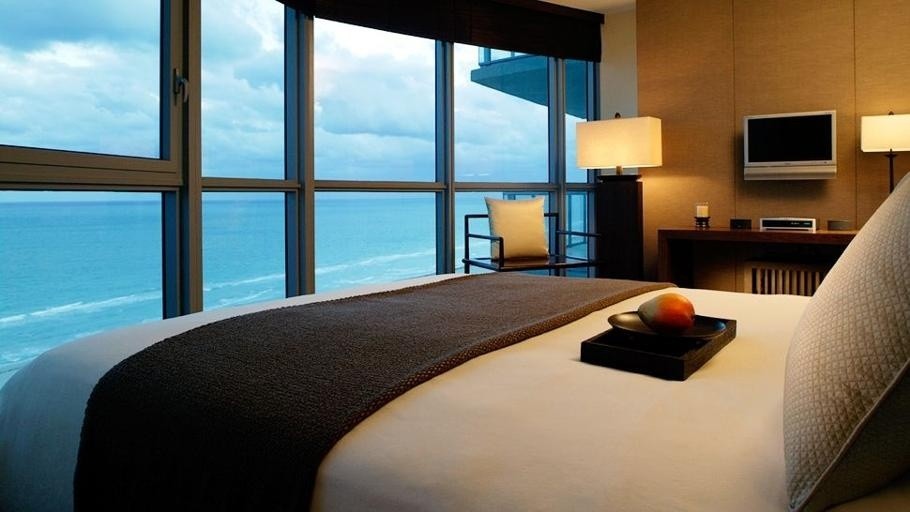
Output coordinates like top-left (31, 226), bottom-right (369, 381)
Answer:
top-left (827, 220), bottom-right (850, 230)
top-left (730, 218), bottom-right (752, 229)
top-left (744, 166), bottom-right (837, 180)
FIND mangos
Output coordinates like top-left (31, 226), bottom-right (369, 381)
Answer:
top-left (637, 291), bottom-right (695, 333)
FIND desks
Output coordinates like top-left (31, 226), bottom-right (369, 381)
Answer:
top-left (656, 226), bottom-right (860, 282)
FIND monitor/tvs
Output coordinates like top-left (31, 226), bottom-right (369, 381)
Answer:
top-left (742, 110), bottom-right (837, 167)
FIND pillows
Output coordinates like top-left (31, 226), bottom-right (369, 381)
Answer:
top-left (485, 196), bottom-right (551, 262)
top-left (783, 170), bottom-right (910, 510)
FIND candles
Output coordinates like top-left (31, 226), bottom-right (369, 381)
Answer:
top-left (697, 205), bottom-right (708, 218)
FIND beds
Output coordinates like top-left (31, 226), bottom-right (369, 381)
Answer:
top-left (1, 273), bottom-right (910, 511)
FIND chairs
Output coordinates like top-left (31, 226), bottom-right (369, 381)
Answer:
top-left (462, 213), bottom-right (603, 281)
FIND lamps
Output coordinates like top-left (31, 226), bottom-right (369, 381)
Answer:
top-left (575, 112), bottom-right (662, 181)
top-left (860, 111), bottom-right (910, 194)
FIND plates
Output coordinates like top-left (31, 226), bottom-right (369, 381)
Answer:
top-left (608, 309), bottom-right (727, 341)
